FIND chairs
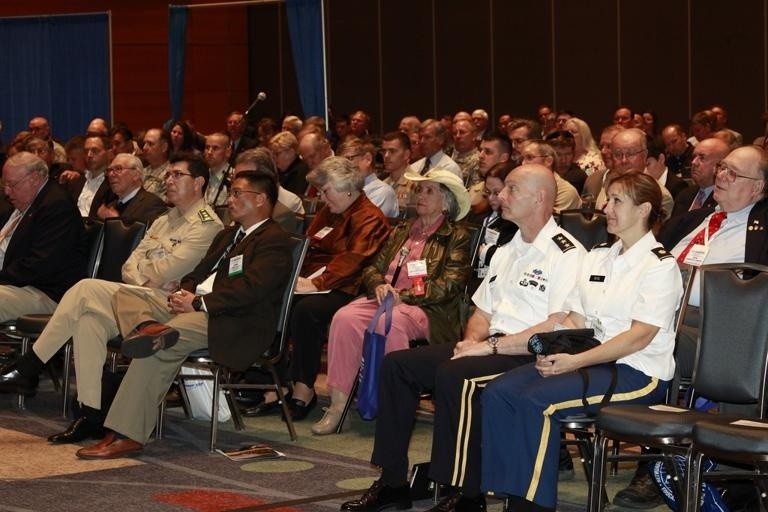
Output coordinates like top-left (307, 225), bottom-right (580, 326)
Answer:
top-left (558, 208), bottom-right (767, 511)
top-left (0, 195), bottom-right (313, 455)
top-left (392, 207), bottom-right (482, 272)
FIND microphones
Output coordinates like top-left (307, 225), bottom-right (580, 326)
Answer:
top-left (244, 91), bottom-right (267, 115)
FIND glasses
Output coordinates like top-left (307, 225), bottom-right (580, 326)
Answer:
top-left (714, 163), bottom-right (759, 184)
top-left (344, 153), bottom-right (362, 161)
top-left (0, 171), bottom-right (32, 193)
top-left (519, 154), bottom-right (549, 163)
top-left (612, 149), bottom-right (644, 159)
top-left (226, 189), bottom-right (261, 199)
top-left (103, 165), bottom-right (136, 177)
top-left (163, 171), bottom-right (193, 180)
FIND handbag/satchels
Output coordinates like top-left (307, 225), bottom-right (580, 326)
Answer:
top-left (356, 289), bottom-right (394, 421)
top-left (527, 327), bottom-right (620, 418)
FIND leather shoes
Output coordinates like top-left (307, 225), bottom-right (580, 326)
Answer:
top-left (75, 430), bottom-right (145, 462)
top-left (47, 410), bottom-right (105, 445)
top-left (2, 365), bottom-right (41, 399)
top-left (611, 446), bottom-right (667, 511)
top-left (240, 391), bottom-right (292, 418)
top-left (120, 319), bottom-right (180, 360)
top-left (422, 492), bottom-right (488, 512)
top-left (339, 479), bottom-right (414, 512)
top-left (281, 390), bottom-right (318, 423)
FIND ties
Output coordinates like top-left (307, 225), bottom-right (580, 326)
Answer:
top-left (0, 211), bottom-right (23, 245)
top-left (676, 211), bottom-right (728, 265)
top-left (196, 230), bottom-right (246, 286)
top-left (689, 190), bottom-right (706, 212)
top-left (420, 158), bottom-right (431, 176)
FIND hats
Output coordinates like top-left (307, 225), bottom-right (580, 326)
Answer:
top-left (403, 166), bottom-right (472, 223)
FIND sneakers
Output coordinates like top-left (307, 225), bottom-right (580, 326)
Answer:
top-left (311, 405), bottom-right (353, 436)
top-left (163, 385), bottom-right (183, 406)
top-left (556, 449), bottom-right (575, 482)
